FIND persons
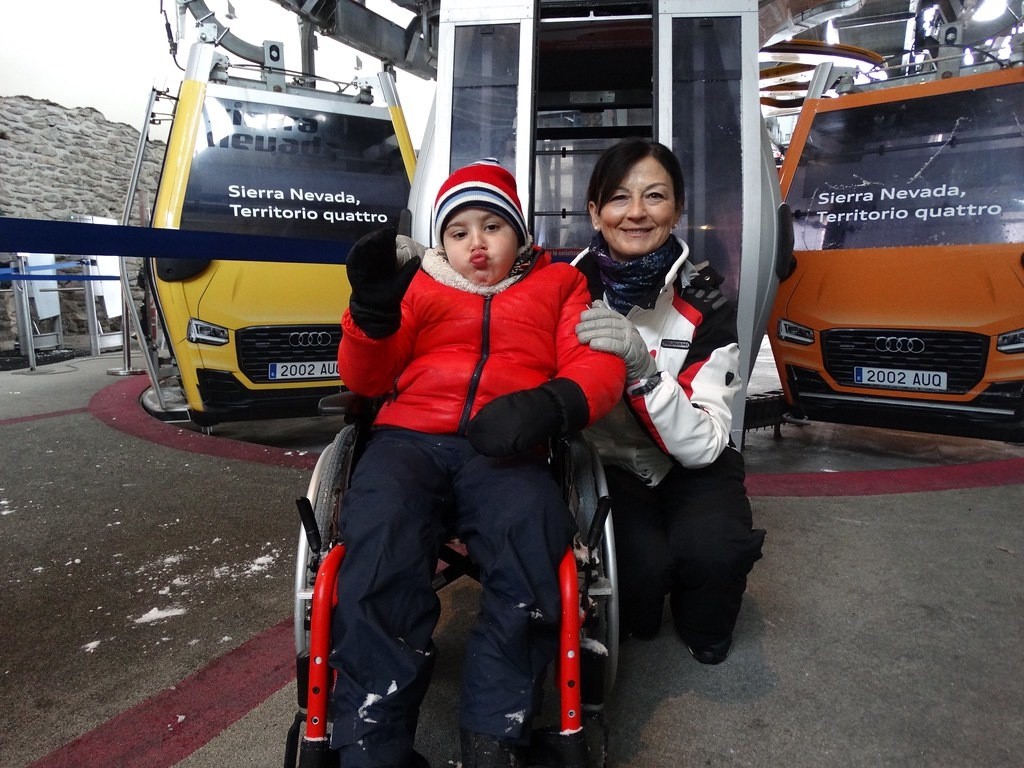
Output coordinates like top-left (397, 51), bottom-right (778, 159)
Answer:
top-left (567, 139), bottom-right (767, 665)
top-left (325, 156), bottom-right (625, 768)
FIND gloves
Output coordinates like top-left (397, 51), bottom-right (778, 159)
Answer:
top-left (467, 378), bottom-right (590, 457)
top-left (576, 299), bottom-right (656, 384)
top-left (346, 226), bottom-right (422, 339)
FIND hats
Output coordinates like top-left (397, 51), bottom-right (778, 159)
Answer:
top-left (433, 157), bottom-right (528, 251)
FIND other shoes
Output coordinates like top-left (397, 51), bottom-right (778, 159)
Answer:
top-left (626, 596), bottom-right (664, 640)
top-left (687, 631), bottom-right (732, 664)
top-left (460, 727), bottom-right (522, 768)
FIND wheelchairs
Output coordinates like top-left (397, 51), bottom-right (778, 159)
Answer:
top-left (281, 390), bottom-right (624, 768)
